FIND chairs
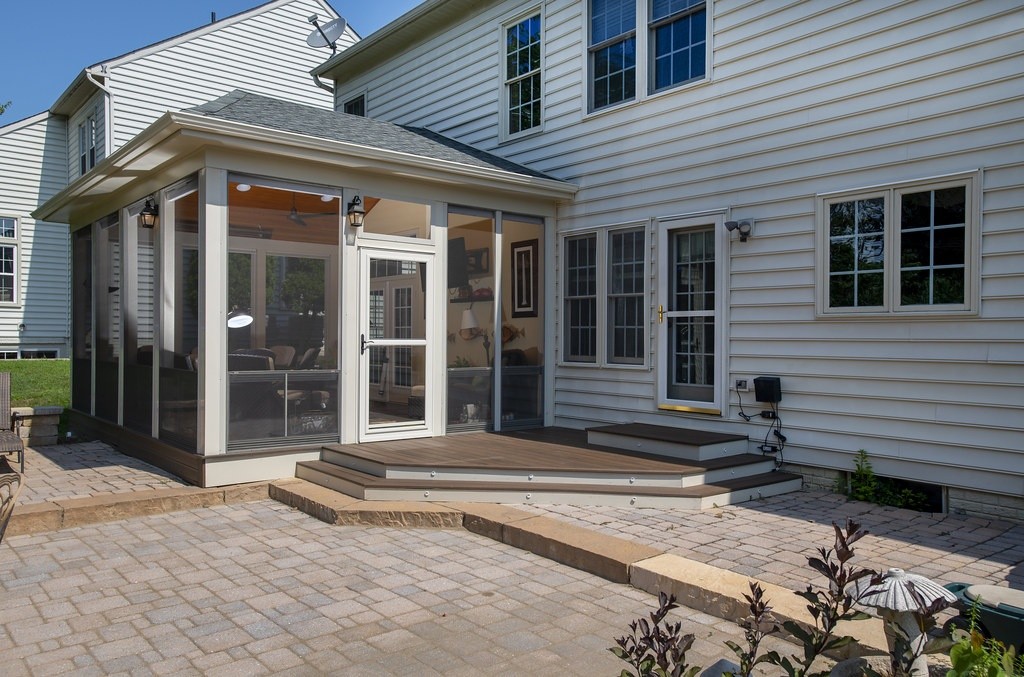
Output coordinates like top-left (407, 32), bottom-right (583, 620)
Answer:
top-left (133, 337), bottom-right (330, 417)
top-left (0, 471), bottom-right (25, 544)
top-left (0, 372), bottom-right (25, 475)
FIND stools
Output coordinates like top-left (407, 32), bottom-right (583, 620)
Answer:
top-left (408, 396), bottom-right (425, 420)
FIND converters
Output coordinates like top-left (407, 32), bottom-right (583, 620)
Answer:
top-left (761, 411), bottom-right (777, 419)
top-left (760, 444), bottom-right (777, 453)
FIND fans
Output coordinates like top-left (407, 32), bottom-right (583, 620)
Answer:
top-left (259, 192), bottom-right (337, 227)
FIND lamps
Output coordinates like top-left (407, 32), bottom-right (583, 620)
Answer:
top-left (227, 299), bottom-right (255, 328)
top-left (139, 196), bottom-right (160, 229)
top-left (348, 194), bottom-right (366, 227)
top-left (460, 307), bottom-right (492, 368)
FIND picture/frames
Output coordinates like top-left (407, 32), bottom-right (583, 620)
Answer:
top-left (509, 237), bottom-right (539, 319)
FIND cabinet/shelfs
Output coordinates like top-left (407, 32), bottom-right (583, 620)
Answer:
top-left (449, 294), bottom-right (493, 303)
